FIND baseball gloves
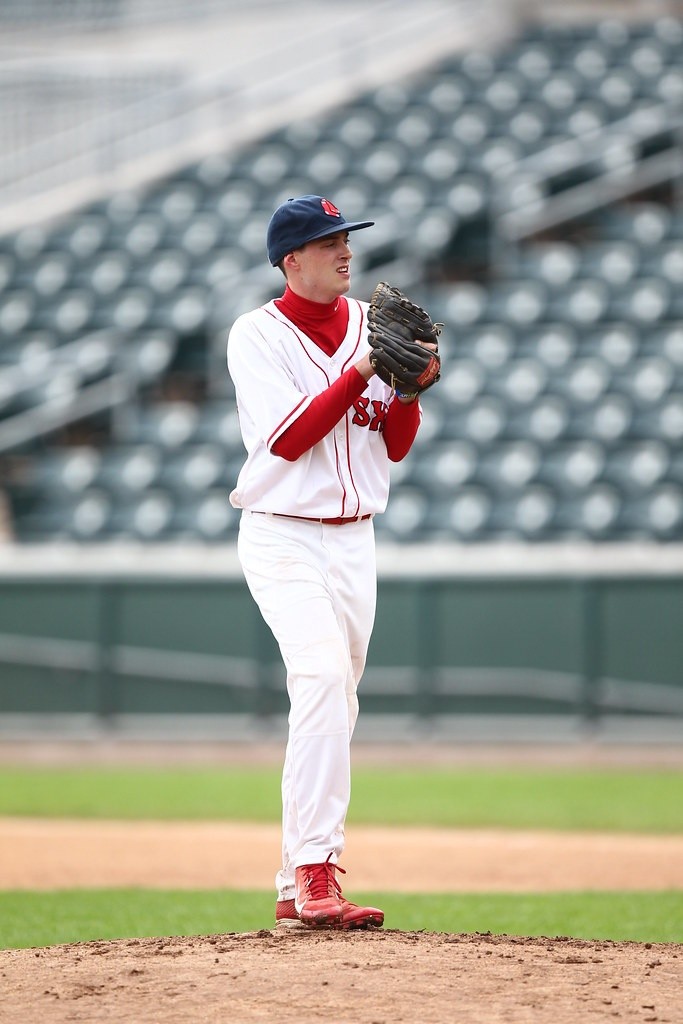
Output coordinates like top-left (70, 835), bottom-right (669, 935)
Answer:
top-left (362, 281), bottom-right (446, 402)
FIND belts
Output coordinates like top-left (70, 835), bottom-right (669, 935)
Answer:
top-left (252, 511), bottom-right (374, 525)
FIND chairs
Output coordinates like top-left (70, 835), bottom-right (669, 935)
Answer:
top-left (0, 25), bottom-right (681, 549)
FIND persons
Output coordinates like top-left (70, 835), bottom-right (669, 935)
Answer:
top-left (226, 192), bottom-right (440, 929)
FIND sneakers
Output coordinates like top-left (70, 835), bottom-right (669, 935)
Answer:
top-left (294, 863), bottom-right (383, 929)
top-left (274, 898), bottom-right (314, 934)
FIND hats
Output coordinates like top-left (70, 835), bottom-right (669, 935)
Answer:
top-left (266, 194), bottom-right (375, 267)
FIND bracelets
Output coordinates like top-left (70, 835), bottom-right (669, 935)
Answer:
top-left (396, 390), bottom-right (419, 398)
